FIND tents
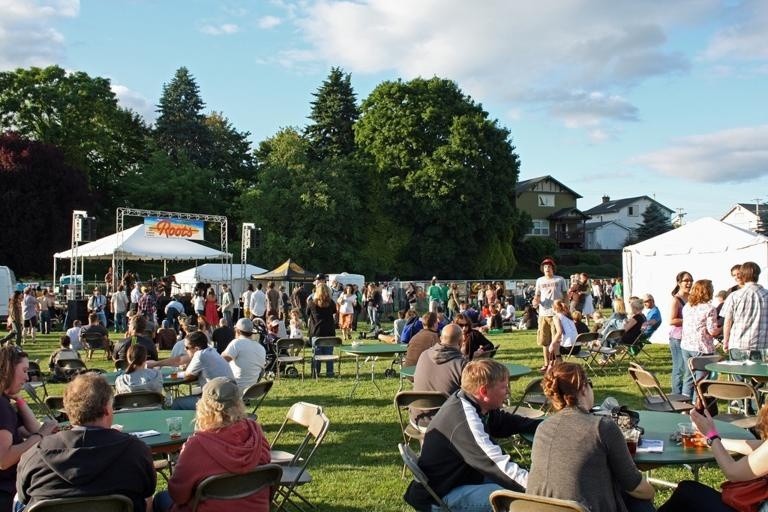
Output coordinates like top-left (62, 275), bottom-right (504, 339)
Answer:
top-left (324, 272), bottom-right (364, 304)
top-left (252, 257), bottom-right (327, 298)
top-left (172, 264), bottom-right (268, 310)
top-left (622, 218), bottom-right (767, 345)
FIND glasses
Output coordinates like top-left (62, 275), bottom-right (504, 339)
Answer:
top-left (457, 323), bottom-right (470, 327)
top-left (643, 298), bottom-right (651, 303)
top-left (9, 345), bottom-right (24, 356)
top-left (681, 279), bottom-right (693, 282)
top-left (583, 378), bottom-right (594, 389)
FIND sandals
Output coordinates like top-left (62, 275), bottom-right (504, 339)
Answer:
top-left (347, 335), bottom-right (353, 340)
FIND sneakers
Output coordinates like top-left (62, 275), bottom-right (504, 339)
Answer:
top-left (541, 364), bottom-right (547, 372)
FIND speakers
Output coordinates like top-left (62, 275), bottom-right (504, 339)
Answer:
top-left (76, 217), bottom-right (98, 243)
top-left (246, 228), bottom-right (261, 249)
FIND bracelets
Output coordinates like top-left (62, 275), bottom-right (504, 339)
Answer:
top-left (31, 431), bottom-right (44, 438)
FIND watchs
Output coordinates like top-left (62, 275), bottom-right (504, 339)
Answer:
top-left (706, 435), bottom-right (721, 445)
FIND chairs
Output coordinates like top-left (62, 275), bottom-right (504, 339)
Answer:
top-left (276, 338), bottom-right (305, 384)
top-left (618, 330), bottom-right (644, 366)
top-left (26, 496), bottom-right (134, 512)
top-left (269, 402), bottom-right (322, 500)
top-left (490, 490), bottom-right (589, 512)
top-left (761, 501), bottom-right (767, 510)
top-left (730, 349), bottom-right (741, 360)
top-left (567, 333), bottom-right (609, 377)
top-left (242, 380), bottom-right (273, 421)
top-left (24, 383), bottom-right (69, 423)
top-left (313, 337), bottom-right (342, 382)
top-left (82, 333), bottom-right (111, 361)
top-left (271, 413), bottom-right (330, 512)
top-left (589, 330), bottom-right (625, 376)
top-left (630, 362), bottom-right (692, 403)
top-left (750, 351), bottom-right (762, 360)
top-left (697, 380), bottom-right (759, 416)
top-left (642, 339), bottom-right (652, 359)
top-left (115, 359), bottom-right (128, 371)
top-left (113, 392), bottom-right (173, 481)
top-left (395, 392), bottom-right (449, 480)
top-left (398, 442), bottom-right (451, 511)
top-left (192, 464), bottom-right (283, 512)
top-left (688, 355), bottom-right (725, 413)
top-left (628, 367), bottom-right (694, 411)
top-left (56, 359), bottom-right (87, 377)
top-left (500, 377), bottom-right (549, 464)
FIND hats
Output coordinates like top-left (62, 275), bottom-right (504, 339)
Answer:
top-left (542, 258), bottom-right (555, 266)
top-left (234, 317), bottom-right (254, 334)
top-left (202, 376), bottom-right (241, 411)
top-left (314, 272), bottom-right (326, 281)
top-left (272, 320), bottom-right (279, 326)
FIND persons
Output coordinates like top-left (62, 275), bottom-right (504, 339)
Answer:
top-left (0, 282), bottom-right (58, 351)
top-left (49, 337), bottom-right (81, 383)
top-left (212, 318), bottom-right (235, 354)
top-left (668, 271), bottom-right (693, 394)
top-left (12, 372), bottom-right (157, 512)
top-left (289, 309), bottom-right (305, 356)
top-left (66, 320), bottom-right (85, 350)
top-left (453, 313), bottom-right (494, 362)
top-left (171, 315), bottom-right (198, 356)
top-left (718, 263), bottom-right (767, 438)
top-left (587, 311), bottom-right (606, 347)
top-left (680, 280), bottom-right (723, 414)
top-left (378, 310), bottom-right (407, 344)
top-left (531, 260), bottom-right (569, 370)
top-left (87, 287), bottom-right (107, 328)
top-left (525, 361), bottom-right (656, 512)
top-left (403, 358), bottom-right (543, 512)
top-left (79, 313), bottom-right (113, 361)
top-left (163, 276), bottom-right (306, 318)
top-left (331, 280), bottom-right (382, 340)
top-left (400, 312), bottom-right (423, 344)
top-left (0, 345), bottom-right (60, 512)
top-left (726, 265), bottom-right (743, 297)
top-left (640, 296), bottom-right (662, 340)
top-left (196, 316), bottom-right (213, 345)
top-left (549, 299), bottom-right (582, 363)
top-left (147, 331), bottom-right (236, 409)
top-left (404, 312), bottom-right (440, 382)
top-left (220, 317), bottom-right (266, 396)
top-left (381, 276), bottom-right (448, 317)
top-left (715, 291), bottom-right (727, 328)
top-left (114, 345), bottom-right (166, 410)
top-left (568, 272), bottom-right (623, 315)
top-left (599, 299), bottom-right (647, 366)
top-left (657, 401), bottom-right (768, 512)
top-left (571, 311), bottom-right (589, 334)
top-left (408, 324), bottom-right (469, 451)
top-left (446, 279), bottom-right (539, 324)
top-left (152, 376), bottom-right (271, 511)
top-left (585, 298), bottom-right (628, 348)
top-left (106, 268), bottom-right (164, 363)
top-left (306, 283), bottom-right (340, 377)
top-left (263, 319), bottom-right (289, 380)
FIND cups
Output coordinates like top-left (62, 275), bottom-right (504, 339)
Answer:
top-left (677, 421), bottom-right (697, 450)
top-left (167, 416), bottom-right (183, 441)
top-left (170, 371), bottom-right (178, 381)
